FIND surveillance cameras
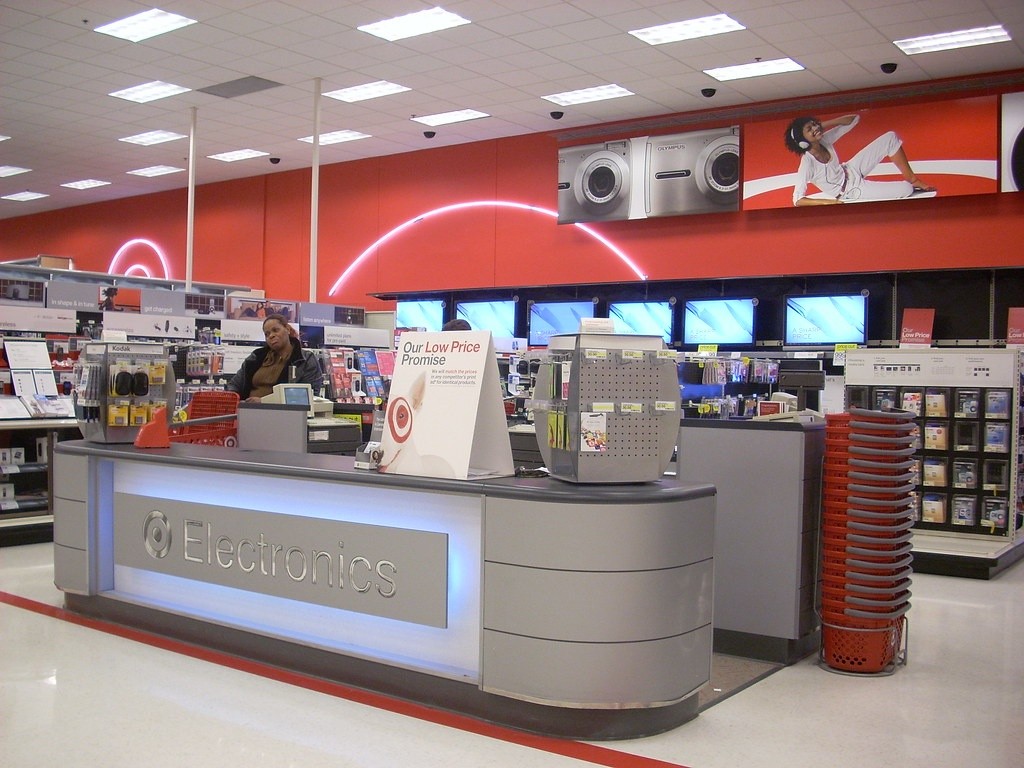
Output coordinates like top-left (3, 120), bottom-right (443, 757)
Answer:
top-left (701, 88), bottom-right (716, 97)
top-left (881, 63), bottom-right (897, 74)
top-left (550, 112), bottom-right (563, 119)
top-left (270, 158), bottom-right (280, 164)
top-left (424, 132), bottom-right (435, 138)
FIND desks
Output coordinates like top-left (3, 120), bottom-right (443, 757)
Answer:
top-left (52, 441), bottom-right (717, 743)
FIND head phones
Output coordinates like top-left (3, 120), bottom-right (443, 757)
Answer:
top-left (791, 128), bottom-right (809, 149)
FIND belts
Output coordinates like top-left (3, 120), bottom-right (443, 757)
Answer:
top-left (835, 165), bottom-right (848, 199)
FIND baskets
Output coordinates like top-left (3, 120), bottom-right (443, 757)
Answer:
top-left (813, 407), bottom-right (917, 678)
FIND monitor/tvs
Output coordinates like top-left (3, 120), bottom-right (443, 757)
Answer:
top-left (454, 299), bottom-right (519, 339)
top-left (273, 384), bottom-right (314, 418)
top-left (682, 296), bottom-right (757, 349)
top-left (527, 299), bottom-right (597, 347)
top-left (783, 291), bottom-right (869, 352)
top-left (607, 300), bottom-right (675, 348)
top-left (396, 299), bottom-right (445, 332)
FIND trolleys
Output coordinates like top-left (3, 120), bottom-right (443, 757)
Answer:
top-left (168, 392), bottom-right (239, 446)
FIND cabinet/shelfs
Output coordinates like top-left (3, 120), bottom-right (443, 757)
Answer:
top-left (675, 418), bottom-right (827, 667)
top-left (0, 418), bottom-right (82, 531)
top-left (306, 426), bottom-right (361, 457)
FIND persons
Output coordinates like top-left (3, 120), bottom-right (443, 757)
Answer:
top-left (99, 287), bottom-right (124, 311)
top-left (226, 313), bottom-right (324, 404)
top-left (239, 302), bottom-right (266, 319)
top-left (784, 114), bottom-right (938, 206)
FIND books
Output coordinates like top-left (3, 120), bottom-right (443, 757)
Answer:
top-left (20, 395), bottom-right (68, 417)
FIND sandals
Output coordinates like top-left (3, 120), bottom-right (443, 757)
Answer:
top-left (910, 179), bottom-right (936, 192)
